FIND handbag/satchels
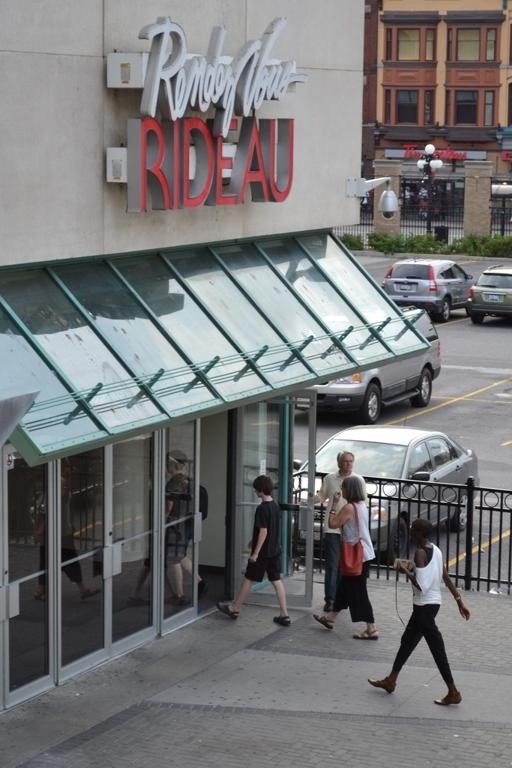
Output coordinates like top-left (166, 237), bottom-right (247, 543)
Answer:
top-left (33, 517), bottom-right (49, 544)
top-left (340, 534), bottom-right (364, 576)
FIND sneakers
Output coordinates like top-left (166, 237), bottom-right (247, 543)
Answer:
top-left (171, 579), bottom-right (207, 605)
top-left (324, 601), bottom-right (334, 611)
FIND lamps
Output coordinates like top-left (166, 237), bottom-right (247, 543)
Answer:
top-left (346, 170), bottom-right (399, 222)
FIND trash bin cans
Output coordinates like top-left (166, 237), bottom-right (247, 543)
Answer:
top-left (434, 226), bottom-right (448, 245)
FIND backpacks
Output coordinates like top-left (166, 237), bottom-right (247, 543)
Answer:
top-left (187, 481), bottom-right (210, 538)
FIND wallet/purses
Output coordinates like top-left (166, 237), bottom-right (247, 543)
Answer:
top-left (393, 558), bottom-right (414, 573)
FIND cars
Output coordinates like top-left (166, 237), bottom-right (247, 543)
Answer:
top-left (293, 423), bottom-right (481, 561)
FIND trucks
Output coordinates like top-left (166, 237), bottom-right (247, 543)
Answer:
top-left (294, 304), bottom-right (442, 424)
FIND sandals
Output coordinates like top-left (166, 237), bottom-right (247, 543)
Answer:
top-left (274, 615), bottom-right (291, 626)
top-left (353, 629), bottom-right (379, 640)
top-left (217, 602), bottom-right (241, 620)
top-left (312, 614), bottom-right (335, 630)
top-left (33, 592), bottom-right (47, 602)
top-left (79, 589), bottom-right (102, 600)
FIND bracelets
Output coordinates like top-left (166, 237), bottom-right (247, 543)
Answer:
top-left (453, 592), bottom-right (461, 600)
top-left (328, 508), bottom-right (337, 515)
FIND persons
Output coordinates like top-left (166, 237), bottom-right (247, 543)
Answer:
top-left (366, 519), bottom-right (470, 705)
top-left (216, 474), bottom-right (291, 627)
top-left (129, 557), bottom-right (151, 603)
top-left (164, 449), bottom-right (210, 605)
top-left (34, 462), bottom-right (101, 600)
top-left (309, 450), bottom-right (367, 612)
top-left (312, 473), bottom-right (380, 640)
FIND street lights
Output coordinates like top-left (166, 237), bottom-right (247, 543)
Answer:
top-left (416, 143), bottom-right (442, 239)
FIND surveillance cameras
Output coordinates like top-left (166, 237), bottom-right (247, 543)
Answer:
top-left (378, 189), bottom-right (399, 220)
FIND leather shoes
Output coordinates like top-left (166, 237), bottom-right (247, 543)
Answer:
top-left (368, 677), bottom-right (396, 693)
top-left (433, 692), bottom-right (461, 706)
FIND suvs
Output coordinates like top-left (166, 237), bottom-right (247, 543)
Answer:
top-left (381, 258), bottom-right (477, 323)
top-left (466, 264), bottom-right (511, 324)
